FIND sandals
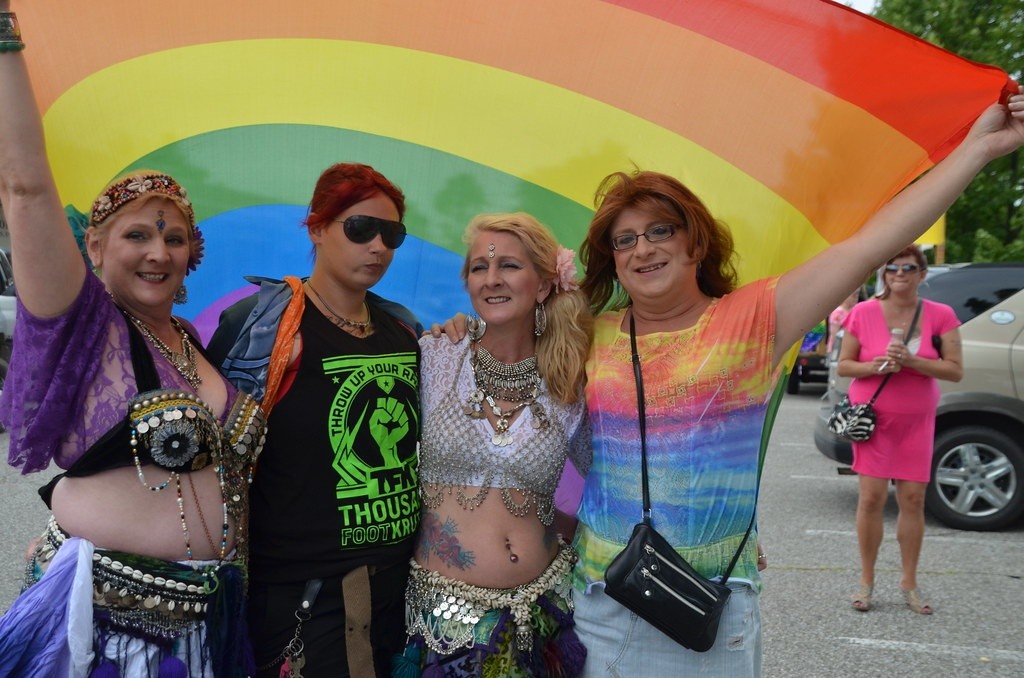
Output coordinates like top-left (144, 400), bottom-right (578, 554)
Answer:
top-left (899, 585), bottom-right (934, 614)
top-left (851, 582), bottom-right (874, 611)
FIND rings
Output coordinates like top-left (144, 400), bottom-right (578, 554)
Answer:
top-left (899, 354), bottom-right (901, 358)
top-left (758, 555), bottom-right (766, 558)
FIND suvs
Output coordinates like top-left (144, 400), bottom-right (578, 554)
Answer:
top-left (814, 259), bottom-right (1024, 532)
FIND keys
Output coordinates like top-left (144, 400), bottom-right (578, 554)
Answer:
top-left (280, 654), bottom-right (306, 678)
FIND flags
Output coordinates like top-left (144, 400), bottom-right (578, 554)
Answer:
top-left (0, 1), bottom-right (1021, 490)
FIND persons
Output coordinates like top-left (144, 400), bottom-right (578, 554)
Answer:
top-left (0, 0), bottom-right (273, 678)
top-left (205, 161), bottom-right (430, 678)
top-left (838, 244), bottom-right (964, 615)
top-left (398, 209), bottom-right (769, 678)
top-left (422, 83), bottom-right (1024, 678)
top-left (826, 288), bottom-right (861, 354)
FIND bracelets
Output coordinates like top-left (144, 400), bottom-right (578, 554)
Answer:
top-left (0, 11), bottom-right (26, 52)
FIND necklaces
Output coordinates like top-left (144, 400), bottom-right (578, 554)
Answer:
top-left (462, 342), bottom-right (551, 446)
top-left (108, 295), bottom-right (203, 392)
top-left (307, 280), bottom-right (371, 335)
top-left (900, 319), bottom-right (909, 327)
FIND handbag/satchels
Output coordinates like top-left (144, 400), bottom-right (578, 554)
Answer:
top-left (603, 516), bottom-right (732, 654)
top-left (827, 395), bottom-right (876, 442)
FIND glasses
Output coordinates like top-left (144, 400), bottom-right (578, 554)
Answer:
top-left (333, 214), bottom-right (408, 250)
top-left (611, 225), bottom-right (681, 251)
top-left (886, 264), bottom-right (917, 274)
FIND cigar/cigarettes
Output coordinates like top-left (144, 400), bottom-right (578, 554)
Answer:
top-left (879, 361), bottom-right (889, 371)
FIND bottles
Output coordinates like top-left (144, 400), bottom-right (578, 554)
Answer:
top-left (887, 328), bottom-right (904, 371)
